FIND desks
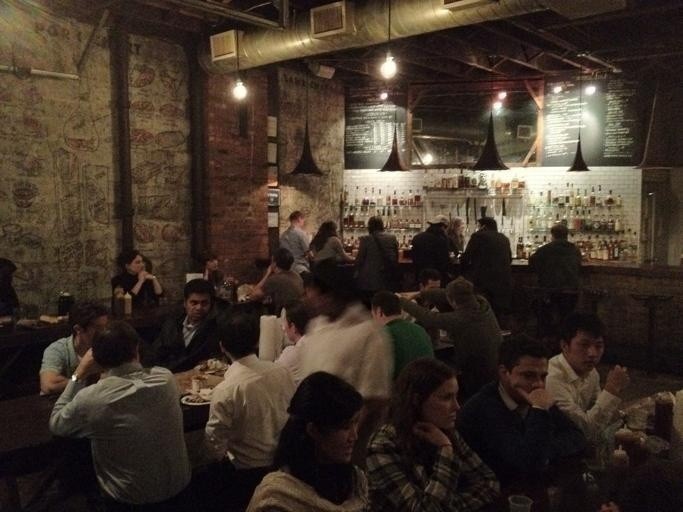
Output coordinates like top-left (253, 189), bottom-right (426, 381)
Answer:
top-left (1, 271), bottom-right (683, 511)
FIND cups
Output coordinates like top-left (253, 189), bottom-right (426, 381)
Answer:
top-left (12, 307), bottom-right (24, 324)
top-left (508, 493), bottom-right (533, 511)
top-left (24, 310), bottom-right (40, 325)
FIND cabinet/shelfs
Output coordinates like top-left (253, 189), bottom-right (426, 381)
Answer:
top-left (340, 199), bottom-right (424, 252)
top-left (529, 197), bottom-right (633, 264)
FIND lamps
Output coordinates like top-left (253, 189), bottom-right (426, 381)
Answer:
top-left (377, 83), bottom-right (411, 175)
top-left (471, 50), bottom-right (509, 172)
top-left (380, 1), bottom-right (398, 80)
top-left (229, 0), bottom-right (247, 100)
top-left (564, 49), bottom-right (592, 173)
top-left (289, 58), bottom-right (324, 178)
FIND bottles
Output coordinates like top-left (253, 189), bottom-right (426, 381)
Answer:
top-left (611, 445), bottom-right (630, 477)
top-left (612, 423), bottom-right (632, 449)
top-left (114, 290), bottom-right (123, 315)
top-left (124, 291), bottom-right (131, 314)
top-left (57, 297), bottom-right (67, 317)
top-left (192, 379), bottom-right (199, 392)
top-left (653, 391), bottom-right (675, 441)
top-left (342, 174), bottom-right (639, 262)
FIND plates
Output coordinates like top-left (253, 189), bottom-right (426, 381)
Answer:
top-left (193, 364), bottom-right (228, 375)
top-left (180, 394), bottom-right (212, 406)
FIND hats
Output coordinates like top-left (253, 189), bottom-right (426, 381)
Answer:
top-left (427, 214), bottom-right (450, 226)
